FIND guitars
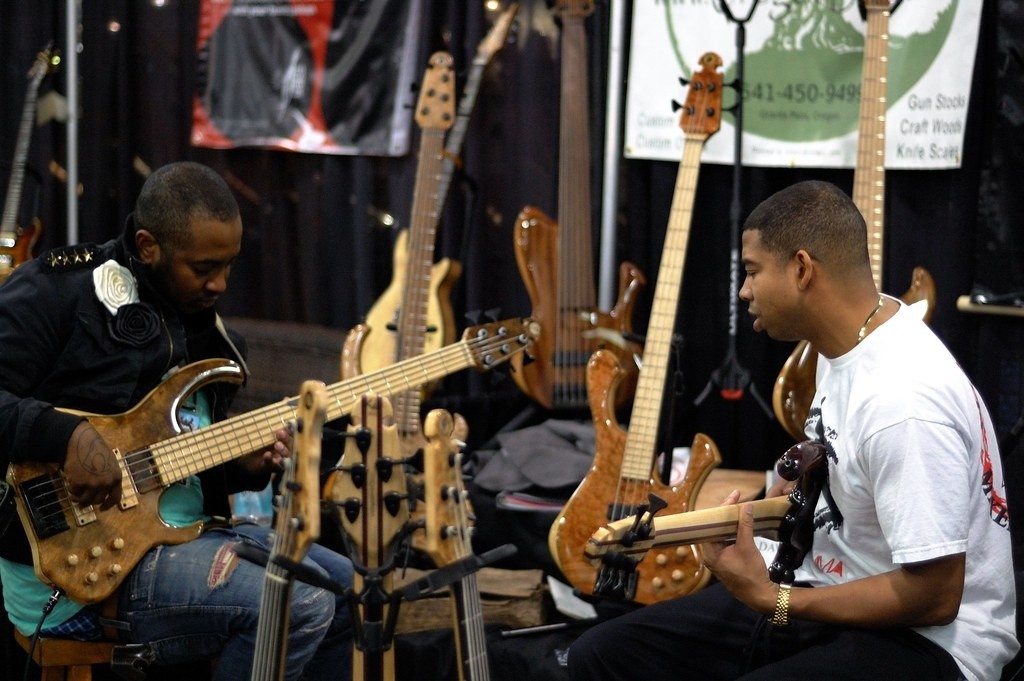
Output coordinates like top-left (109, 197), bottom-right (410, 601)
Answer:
top-left (583, 416), bottom-right (828, 585)
top-left (547, 48), bottom-right (744, 607)
top-left (511, 0), bottom-right (646, 415)
top-left (322, 48), bottom-right (461, 502)
top-left (246, 376), bottom-right (330, 679)
top-left (0, 39), bottom-right (61, 286)
top-left (332, 389), bottom-right (412, 681)
top-left (421, 403), bottom-right (492, 681)
top-left (6, 313), bottom-right (543, 609)
top-left (769, 0), bottom-right (938, 442)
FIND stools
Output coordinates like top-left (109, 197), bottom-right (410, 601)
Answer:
top-left (14, 628), bottom-right (110, 681)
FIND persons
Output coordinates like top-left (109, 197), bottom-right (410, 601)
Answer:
top-left (566, 180), bottom-right (1020, 681)
top-left (0, 163), bottom-right (357, 681)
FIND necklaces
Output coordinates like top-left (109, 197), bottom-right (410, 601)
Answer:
top-left (857, 294), bottom-right (883, 345)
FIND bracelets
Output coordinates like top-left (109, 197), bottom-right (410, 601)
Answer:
top-left (767, 582), bottom-right (792, 627)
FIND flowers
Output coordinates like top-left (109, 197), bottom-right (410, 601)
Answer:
top-left (92, 260), bottom-right (163, 348)
